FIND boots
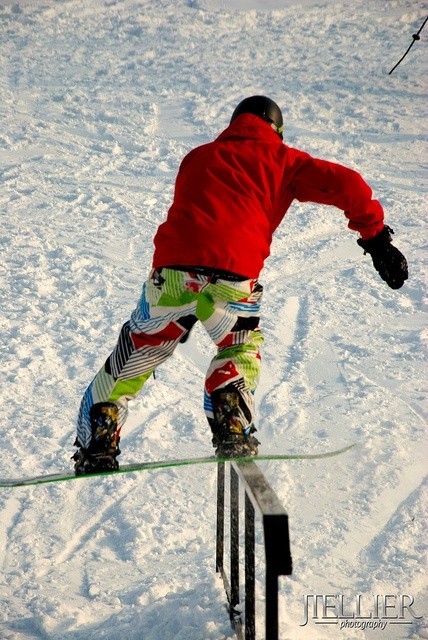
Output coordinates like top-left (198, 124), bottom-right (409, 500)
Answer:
top-left (212, 386), bottom-right (261, 458)
top-left (72, 402), bottom-right (120, 476)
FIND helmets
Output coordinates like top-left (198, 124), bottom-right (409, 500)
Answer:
top-left (231, 96), bottom-right (283, 140)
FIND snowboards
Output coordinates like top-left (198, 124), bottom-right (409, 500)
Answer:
top-left (0, 443), bottom-right (357, 488)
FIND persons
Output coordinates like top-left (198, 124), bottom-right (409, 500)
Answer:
top-left (74, 95), bottom-right (408, 472)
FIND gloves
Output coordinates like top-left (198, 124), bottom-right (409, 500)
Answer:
top-left (357, 225), bottom-right (409, 290)
